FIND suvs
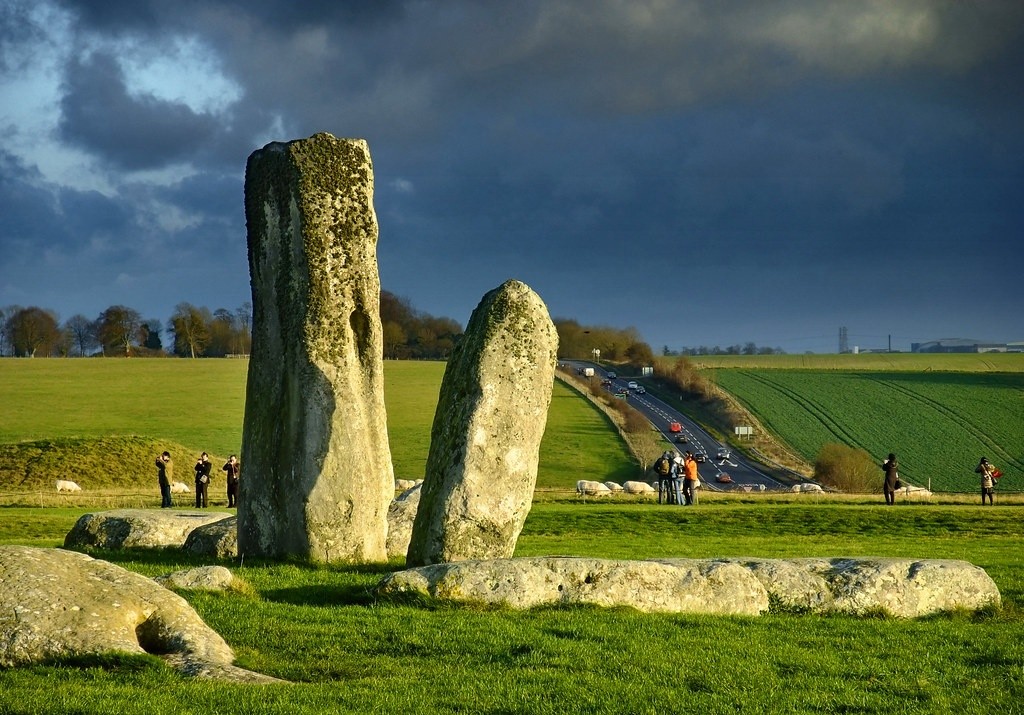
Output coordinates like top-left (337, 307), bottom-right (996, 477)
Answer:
top-left (717, 448), bottom-right (730, 459)
top-left (694, 453), bottom-right (705, 463)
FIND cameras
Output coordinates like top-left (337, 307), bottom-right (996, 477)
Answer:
top-left (230, 460), bottom-right (232, 462)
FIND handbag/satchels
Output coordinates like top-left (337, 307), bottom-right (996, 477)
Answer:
top-left (894, 480), bottom-right (902, 490)
top-left (992, 478), bottom-right (997, 485)
top-left (690, 479), bottom-right (701, 489)
top-left (200, 475), bottom-right (208, 484)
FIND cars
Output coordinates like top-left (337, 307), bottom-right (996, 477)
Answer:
top-left (635, 386), bottom-right (645, 394)
top-left (716, 473), bottom-right (730, 483)
top-left (671, 422), bottom-right (681, 432)
top-left (602, 378), bottom-right (611, 385)
top-left (607, 372), bottom-right (617, 379)
top-left (675, 434), bottom-right (687, 443)
top-left (628, 382), bottom-right (638, 389)
top-left (579, 367), bottom-right (589, 374)
top-left (618, 387), bottom-right (629, 396)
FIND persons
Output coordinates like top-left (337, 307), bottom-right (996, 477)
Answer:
top-left (975, 457), bottom-right (996, 506)
top-left (195, 452), bottom-right (212, 508)
top-left (223, 455), bottom-right (239, 508)
top-left (156, 452), bottom-right (174, 508)
top-left (883, 454), bottom-right (898, 505)
top-left (654, 450), bottom-right (700, 506)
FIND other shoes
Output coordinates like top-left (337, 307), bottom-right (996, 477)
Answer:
top-left (226, 503), bottom-right (238, 508)
top-left (991, 501), bottom-right (994, 506)
top-left (887, 502), bottom-right (890, 505)
top-left (657, 499), bottom-right (693, 506)
top-left (891, 502), bottom-right (894, 505)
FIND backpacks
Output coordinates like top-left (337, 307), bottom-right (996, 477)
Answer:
top-left (673, 459), bottom-right (686, 476)
top-left (659, 458), bottom-right (669, 474)
